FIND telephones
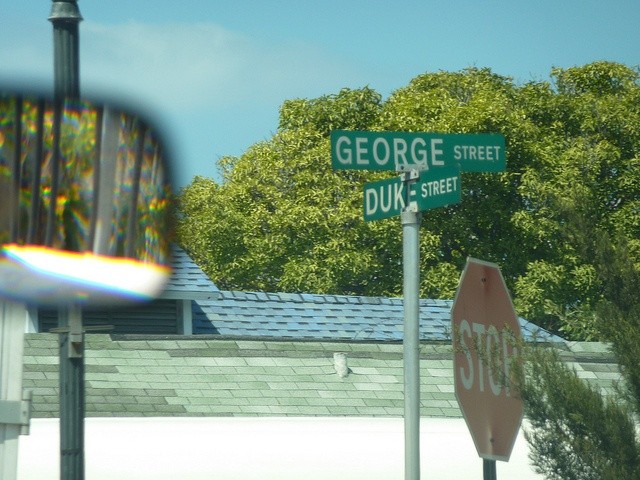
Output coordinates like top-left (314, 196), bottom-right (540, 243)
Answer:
top-left (354, 175), bottom-right (468, 222)
top-left (332, 125), bottom-right (514, 172)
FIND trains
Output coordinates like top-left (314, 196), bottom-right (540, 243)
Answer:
top-left (451, 255), bottom-right (531, 461)
